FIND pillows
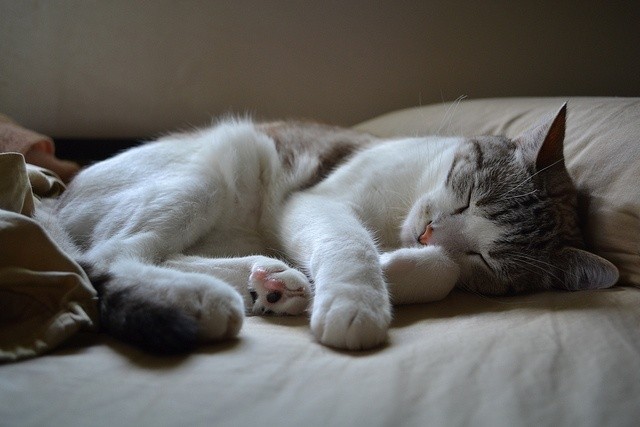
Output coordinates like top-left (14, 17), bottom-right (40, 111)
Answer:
top-left (349, 91), bottom-right (640, 291)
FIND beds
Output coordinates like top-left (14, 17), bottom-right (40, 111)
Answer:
top-left (0, 285), bottom-right (640, 426)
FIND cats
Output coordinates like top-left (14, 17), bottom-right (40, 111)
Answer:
top-left (29, 101), bottom-right (623, 359)
